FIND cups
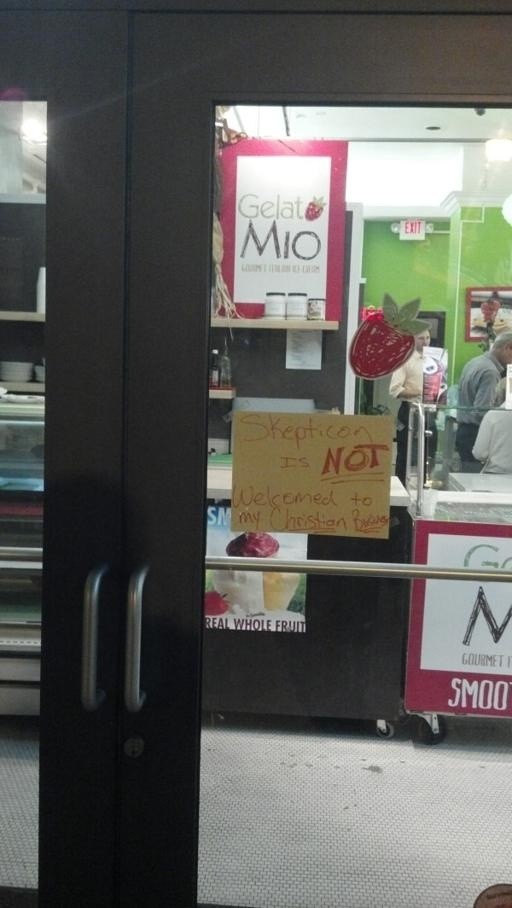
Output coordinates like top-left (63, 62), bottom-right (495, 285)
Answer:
top-left (481, 306), bottom-right (500, 323)
top-left (263, 570), bottom-right (301, 611)
top-left (211, 569), bottom-right (264, 618)
top-left (423, 358), bottom-right (443, 402)
top-left (308, 298), bottom-right (326, 321)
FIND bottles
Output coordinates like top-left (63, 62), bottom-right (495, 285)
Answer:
top-left (286, 292), bottom-right (308, 319)
top-left (264, 291), bottom-right (285, 319)
top-left (209, 349), bottom-right (219, 386)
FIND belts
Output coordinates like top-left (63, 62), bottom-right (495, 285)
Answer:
top-left (401, 401), bottom-right (411, 407)
top-left (456, 421), bottom-right (480, 432)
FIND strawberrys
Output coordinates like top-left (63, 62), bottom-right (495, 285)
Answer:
top-left (203, 591), bottom-right (227, 615)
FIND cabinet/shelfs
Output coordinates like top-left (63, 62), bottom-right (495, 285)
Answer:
top-left (0, 311), bottom-right (339, 399)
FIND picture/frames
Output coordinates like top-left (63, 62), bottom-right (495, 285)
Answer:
top-left (464, 286), bottom-right (512, 342)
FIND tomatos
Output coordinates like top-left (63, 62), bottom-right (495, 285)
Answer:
top-left (350, 294), bottom-right (429, 379)
top-left (306, 197), bottom-right (326, 221)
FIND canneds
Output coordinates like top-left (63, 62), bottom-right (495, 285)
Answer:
top-left (264, 292), bottom-right (326, 321)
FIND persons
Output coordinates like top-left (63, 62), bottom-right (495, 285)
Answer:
top-left (455, 331), bottom-right (512, 472)
top-left (388, 327), bottom-right (442, 489)
top-left (471, 402), bottom-right (512, 477)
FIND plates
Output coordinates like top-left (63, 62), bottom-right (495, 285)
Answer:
top-left (472, 319), bottom-right (509, 329)
top-left (0, 387), bottom-right (45, 404)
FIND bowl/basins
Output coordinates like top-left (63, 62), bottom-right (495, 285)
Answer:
top-left (1, 360), bottom-right (46, 383)
